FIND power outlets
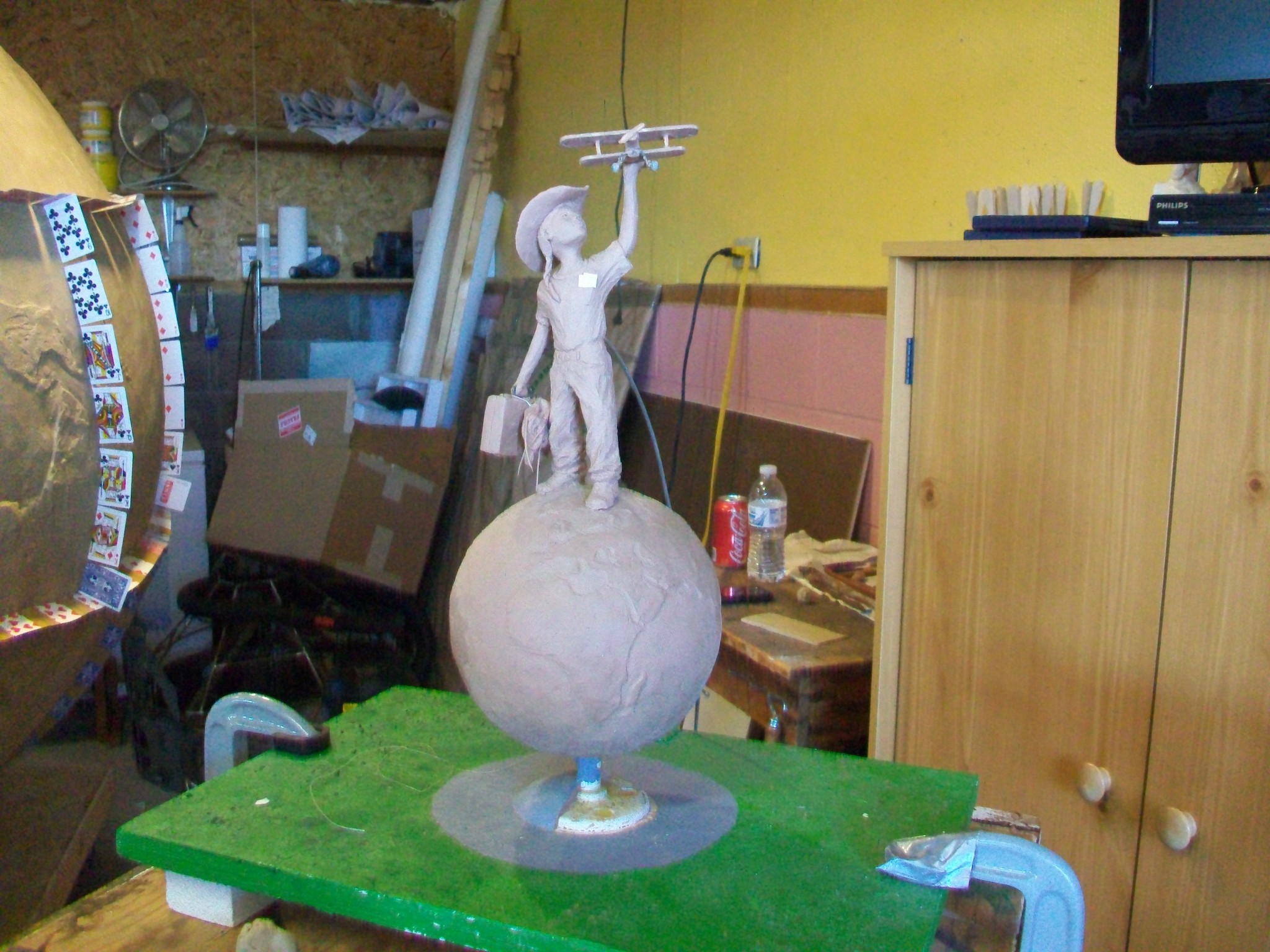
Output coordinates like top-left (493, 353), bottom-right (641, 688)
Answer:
top-left (725, 235), bottom-right (761, 269)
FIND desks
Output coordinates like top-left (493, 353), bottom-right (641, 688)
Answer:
top-left (0, 844), bottom-right (456, 952)
top-left (687, 564), bottom-right (883, 755)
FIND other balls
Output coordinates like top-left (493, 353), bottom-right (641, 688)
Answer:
top-left (446, 483), bottom-right (725, 759)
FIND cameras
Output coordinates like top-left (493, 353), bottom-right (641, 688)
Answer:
top-left (1115, 0), bottom-right (1270, 196)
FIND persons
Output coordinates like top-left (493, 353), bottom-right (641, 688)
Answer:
top-left (511, 160), bottom-right (646, 511)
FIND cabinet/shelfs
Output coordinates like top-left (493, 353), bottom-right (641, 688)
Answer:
top-left (162, 124), bottom-right (448, 356)
top-left (866, 233), bottom-right (1269, 951)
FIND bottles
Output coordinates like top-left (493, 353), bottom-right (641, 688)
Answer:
top-left (746, 464), bottom-right (788, 583)
top-left (256, 223), bottom-right (271, 278)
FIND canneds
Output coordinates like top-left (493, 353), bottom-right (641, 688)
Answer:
top-left (79, 99), bottom-right (120, 192)
top-left (711, 493), bottom-right (750, 569)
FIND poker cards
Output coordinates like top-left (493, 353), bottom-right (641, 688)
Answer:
top-left (0, 189), bottom-right (194, 637)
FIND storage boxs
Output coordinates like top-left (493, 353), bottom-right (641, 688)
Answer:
top-left (318, 416), bottom-right (464, 601)
top-left (200, 372), bottom-right (357, 563)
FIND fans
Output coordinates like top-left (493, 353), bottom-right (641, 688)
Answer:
top-left (117, 78), bottom-right (209, 190)
